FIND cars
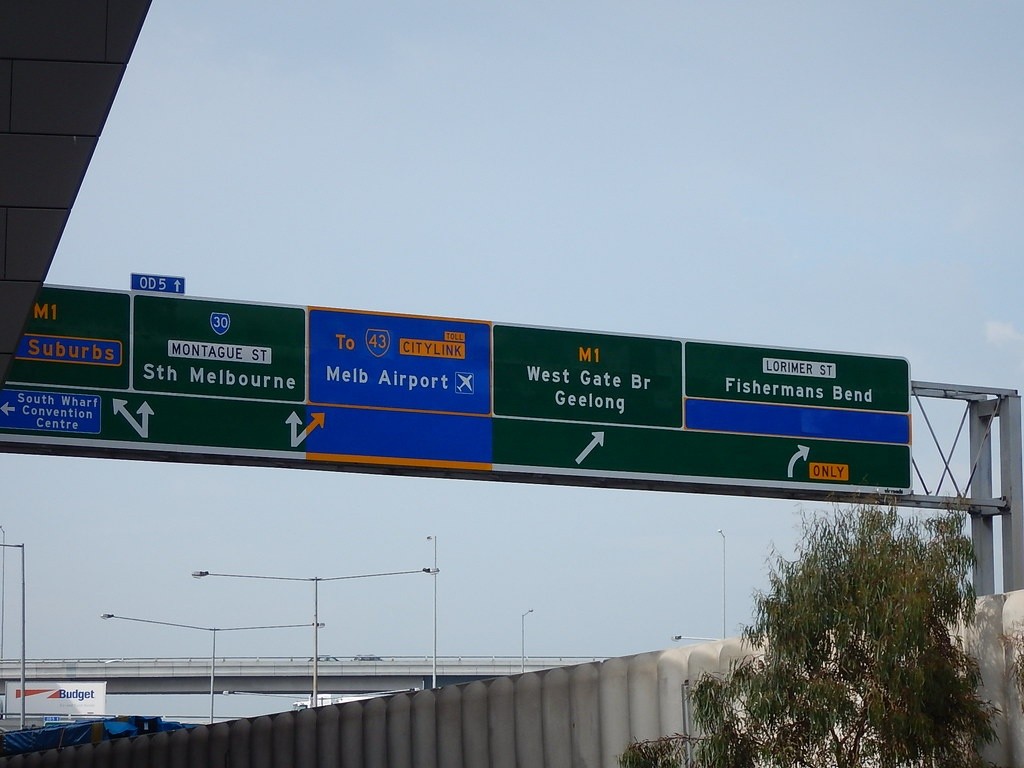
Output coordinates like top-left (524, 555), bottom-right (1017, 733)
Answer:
top-left (307, 654), bottom-right (383, 661)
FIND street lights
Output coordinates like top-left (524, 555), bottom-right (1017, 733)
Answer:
top-left (521, 609), bottom-right (535, 675)
top-left (719, 529), bottom-right (726, 640)
top-left (223, 688), bottom-right (418, 707)
top-left (427, 535), bottom-right (439, 691)
top-left (191, 568), bottom-right (437, 707)
top-left (101, 613), bottom-right (324, 723)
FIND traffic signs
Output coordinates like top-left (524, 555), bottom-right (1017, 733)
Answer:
top-left (0, 283), bottom-right (913, 494)
